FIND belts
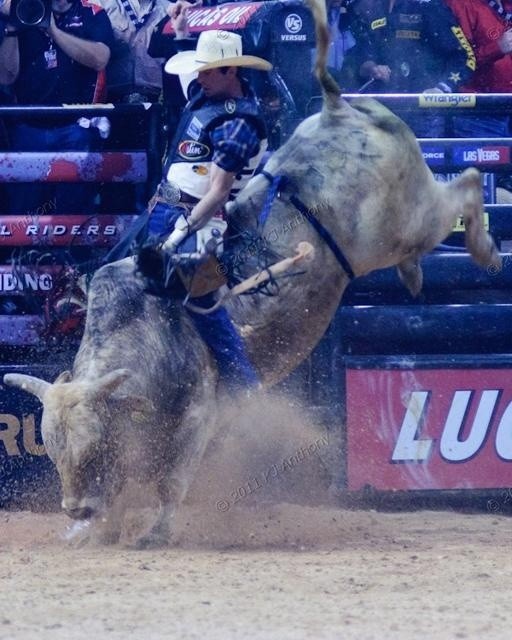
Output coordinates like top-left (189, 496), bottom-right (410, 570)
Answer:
top-left (159, 183), bottom-right (196, 204)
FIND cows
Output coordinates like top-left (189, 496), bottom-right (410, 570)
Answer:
top-left (3, 0), bottom-right (505, 550)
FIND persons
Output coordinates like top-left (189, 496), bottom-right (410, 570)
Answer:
top-left (0, 1), bottom-right (512, 312)
top-left (53, 29), bottom-right (273, 399)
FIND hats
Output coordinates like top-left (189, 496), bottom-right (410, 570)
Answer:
top-left (164, 30), bottom-right (273, 75)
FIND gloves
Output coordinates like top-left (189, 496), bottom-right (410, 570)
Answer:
top-left (156, 215), bottom-right (192, 257)
